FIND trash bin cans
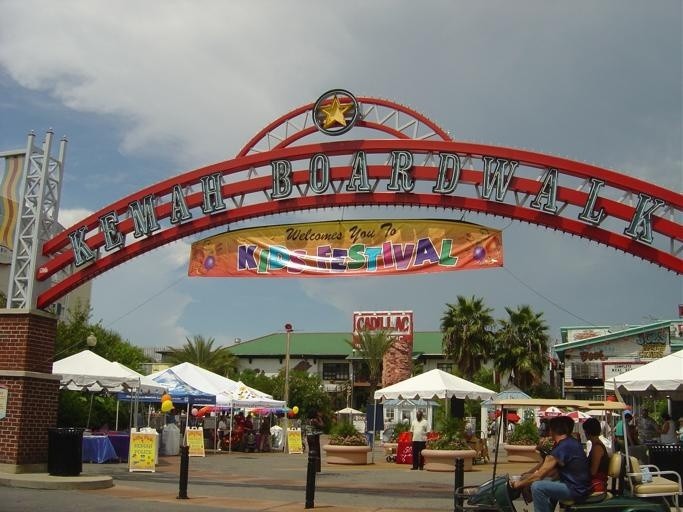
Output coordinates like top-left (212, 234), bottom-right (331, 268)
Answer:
top-left (647, 444), bottom-right (683, 507)
top-left (48, 428), bottom-right (85, 477)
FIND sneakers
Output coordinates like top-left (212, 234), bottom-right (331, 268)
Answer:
top-left (410, 468), bottom-right (424, 470)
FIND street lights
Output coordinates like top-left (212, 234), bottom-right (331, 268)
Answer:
top-left (285, 324), bottom-right (294, 401)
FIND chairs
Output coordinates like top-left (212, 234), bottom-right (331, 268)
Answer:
top-left (624, 456), bottom-right (683, 511)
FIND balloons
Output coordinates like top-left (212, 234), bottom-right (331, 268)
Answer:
top-left (192, 405), bottom-right (286, 418)
top-left (193, 249), bottom-right (215, 271)
top-left (473, 236), bottom-right (500, 262)
top-left (288, 406), bottom-right (302, 419)
top-left (161, 394), bottom-right (173, 412)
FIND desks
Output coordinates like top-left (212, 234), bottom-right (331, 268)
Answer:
top-left (82, 433), bottom-right (129, 466)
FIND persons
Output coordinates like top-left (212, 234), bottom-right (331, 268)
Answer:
top-left (410, 410), bottom-right (430, 470)
top-left (508, 416), bottom-right (608, 512)
top-left (217, 411), bottom-right (253, 451)
top-left (613, 408), bottom-right (683, 450)
top-left (488, 418), bottom-right (496, 452)
top-left (305, 408), bottom-right (330, 472)
top-left (463, 420), bottom-right (472, 432)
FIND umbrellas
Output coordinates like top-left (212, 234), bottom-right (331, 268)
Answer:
top-left (565, 409), bottom-right (620, 423)
top-left (53, 350), bottom-right (166, 429)
